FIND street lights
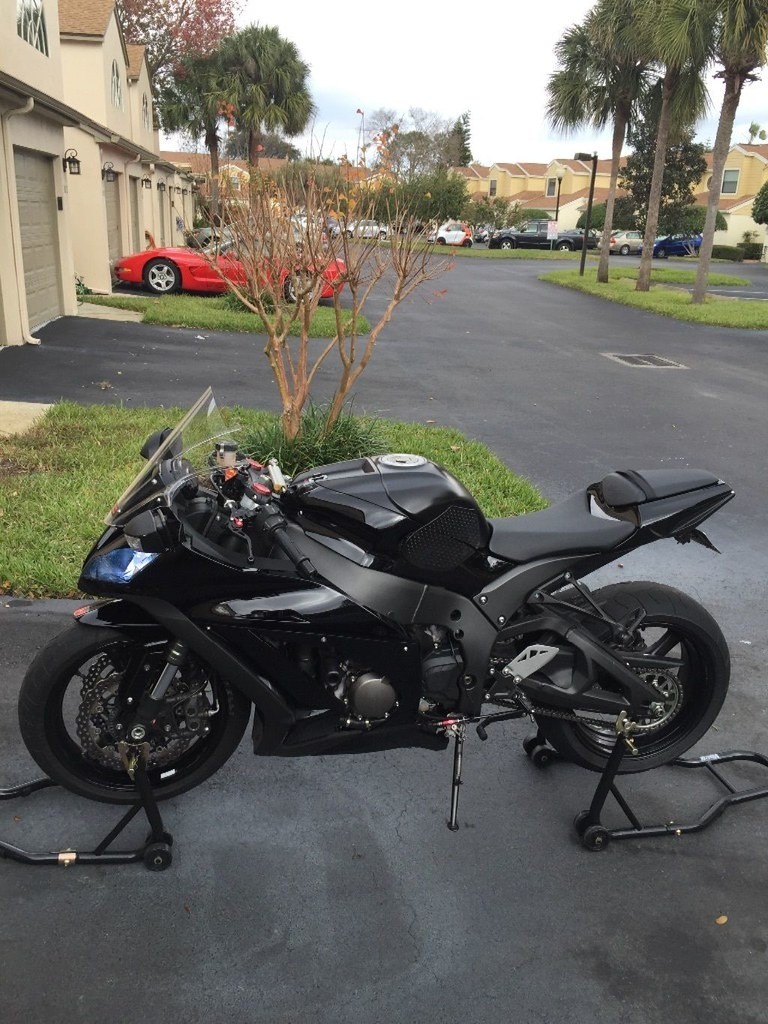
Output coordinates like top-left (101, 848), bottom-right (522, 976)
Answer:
top-left (573, 153), bottom-right (599, 276)
top-left (555, 164), bottom-right (566, 221)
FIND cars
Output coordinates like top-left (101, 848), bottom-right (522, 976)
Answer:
top-left (473, 226), bottom-right (511, 243)
top-left (637, 232), bottom-right (703, 258)
top-left (186, 214), bottom-right (330, 254)
top-left (426, 223), bottom-right (473, 248)
top-left (597, 231), bottom-right (644, 256)
top-left (289, 214), bottom-right (396, 240)
top-left (390, 220), bottom-right (434, 235)
top-left (562, 229), bottom-right (601, 249)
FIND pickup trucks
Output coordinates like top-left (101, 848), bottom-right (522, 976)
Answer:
top-left (486, 220), bottom-right (585, 252)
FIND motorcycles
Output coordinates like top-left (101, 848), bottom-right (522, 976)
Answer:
top-left (18, 380), bottom-right (731, 808)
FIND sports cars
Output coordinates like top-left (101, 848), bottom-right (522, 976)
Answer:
top-left (116, 235), bottom-right (348, 305)
